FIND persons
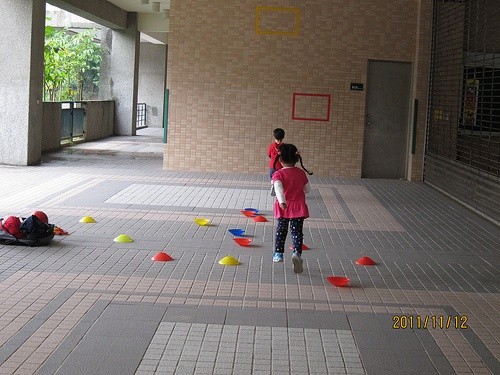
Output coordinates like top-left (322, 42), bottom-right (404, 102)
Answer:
top-left (271, 143), bottom-right (314, 275)
top-left (267, 128), bottom-right (288, 196)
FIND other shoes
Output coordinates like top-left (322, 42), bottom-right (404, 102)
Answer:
top-left (292, 252), bottom-right (303, 274)
top-left (270, 185), bottom-right (276, 196)
top-left (273, 253), bottom-right (283, 262)
